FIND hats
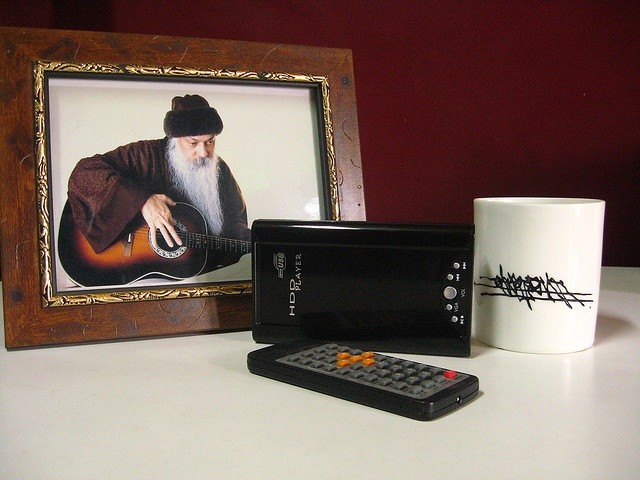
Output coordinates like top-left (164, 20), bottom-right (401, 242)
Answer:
top-left (162, 94), bottom-right (225, 139)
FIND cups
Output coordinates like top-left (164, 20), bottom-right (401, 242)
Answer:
top-left (471, 194), bottom-right (606, 354)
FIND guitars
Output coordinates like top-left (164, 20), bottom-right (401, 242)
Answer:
top-left (57, 199), bottom-right (251, 288)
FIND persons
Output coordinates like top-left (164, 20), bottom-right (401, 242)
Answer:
top-left (68, 94), bottom-right (251, 269)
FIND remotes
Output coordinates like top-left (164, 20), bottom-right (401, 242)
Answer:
top-left (246, 335), bottom-right (480, 422)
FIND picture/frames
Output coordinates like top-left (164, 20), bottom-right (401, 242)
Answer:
top-left (0, 24), bottom-right (368, 350)
